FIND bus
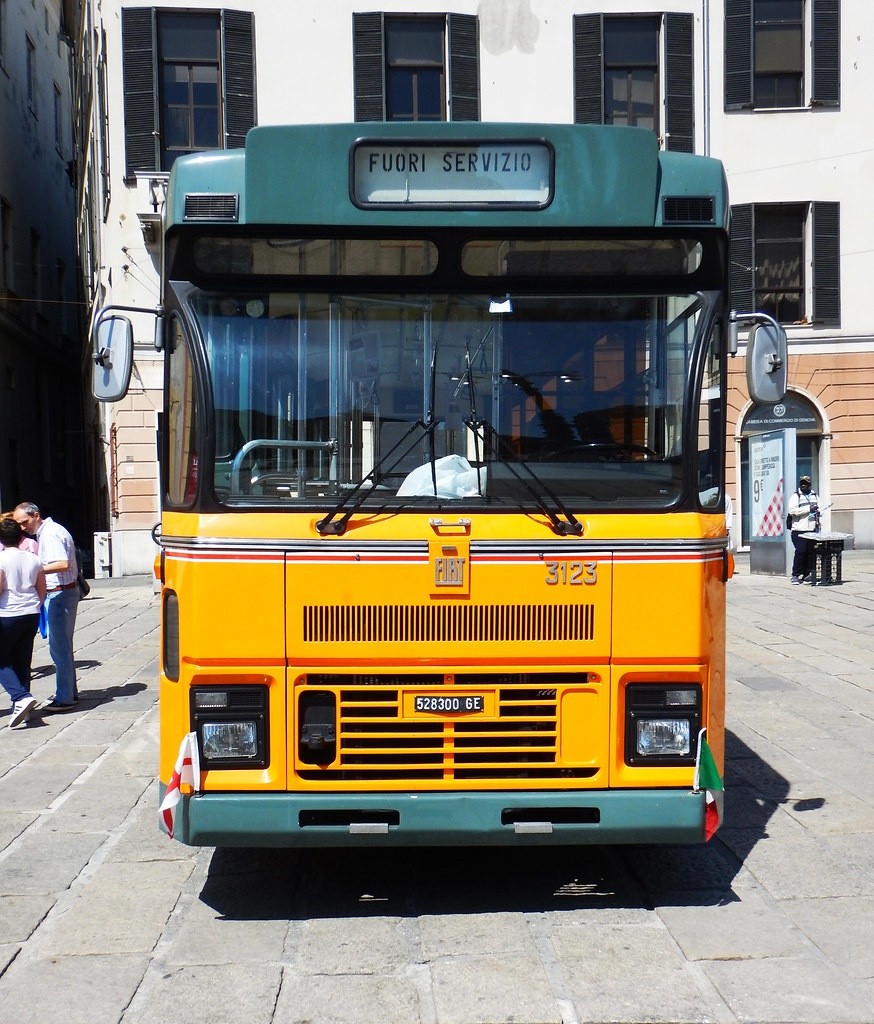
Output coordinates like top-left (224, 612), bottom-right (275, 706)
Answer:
top-left (91, 123), bottom-right (788, 848)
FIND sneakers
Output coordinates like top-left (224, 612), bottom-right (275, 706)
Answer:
top-left (791, 576), bottom-right (799, 584)
top-left (802, 576), bottom-right (812, 584)
top-left (9, 697), bottom-right (37, 727)
top-left (42, 695), bottom-right (80, 711)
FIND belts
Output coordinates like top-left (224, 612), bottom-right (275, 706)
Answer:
top-left (47, 582), bottom-right (75, 592)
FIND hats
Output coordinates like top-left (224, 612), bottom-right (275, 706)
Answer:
top-left (799, 476), bottom-right (811, 482)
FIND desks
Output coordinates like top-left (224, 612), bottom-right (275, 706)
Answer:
top-left (799, 532), bottom-right (855, 585)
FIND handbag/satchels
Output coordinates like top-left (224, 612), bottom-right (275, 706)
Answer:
top-left (77, 572), bottom-right (90, 601)
top-left (40, 596), bottom-right (48, 639)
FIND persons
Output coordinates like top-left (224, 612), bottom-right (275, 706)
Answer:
top-left (0, 500), bottom-right (84, 727)
top-left (789, 475), bottom-right (823, 586)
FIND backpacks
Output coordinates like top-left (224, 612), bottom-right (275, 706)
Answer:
top-left (787, 492), bottom-right (816, 530)
